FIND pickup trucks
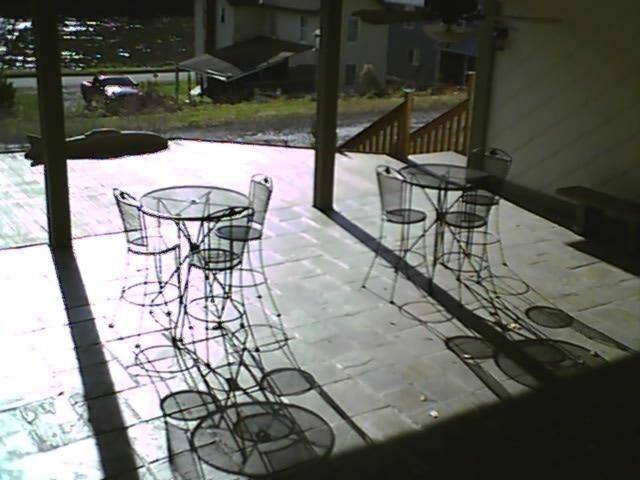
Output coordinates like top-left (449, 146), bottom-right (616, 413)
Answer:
top-left (80, 73), bottom-right (144, 104)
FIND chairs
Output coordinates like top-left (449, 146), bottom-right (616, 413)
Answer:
top-left (112, 172), bottom-right (284, 358)
top-left (131, 322), bottom-right (371, 479)
top-left (363, 143), bottom-right (513, 307)
top-left (404, 276), bottom-right (635, 399)
